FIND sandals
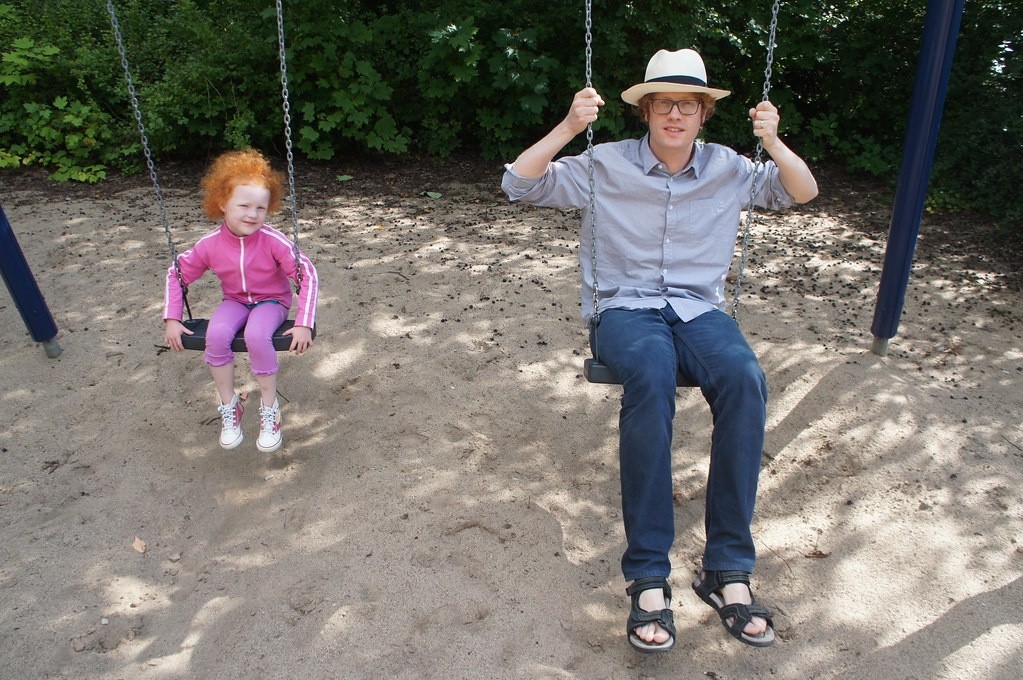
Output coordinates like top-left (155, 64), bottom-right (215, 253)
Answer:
top-left (625, 576), bottom-right (677, 653)
top-left (690, 569), bottom-right (776, 646)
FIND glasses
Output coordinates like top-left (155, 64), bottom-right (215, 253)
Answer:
top-left (650, 96), bottom-right (704, 115)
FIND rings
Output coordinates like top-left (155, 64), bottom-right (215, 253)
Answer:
top-left (760, 120), bottom-right (764, 128)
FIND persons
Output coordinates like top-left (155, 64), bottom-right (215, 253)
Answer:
top-left (501, 49), bottom-right (819, 653)
top-left (163, 153), bottom-right (317, 452)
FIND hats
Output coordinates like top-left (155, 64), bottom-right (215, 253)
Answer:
top-left (621, 48), bottom-right (732, 106)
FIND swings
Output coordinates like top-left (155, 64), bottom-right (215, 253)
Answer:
top-left (106, 7), bottom-right (316, 353)
top-left (585, 1), bottom-right (781, 386)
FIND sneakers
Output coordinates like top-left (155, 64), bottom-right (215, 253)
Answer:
top-left (257, 397), bottom-right (284, 453)
top-left (219, 391), bottom-right (244, 450)
